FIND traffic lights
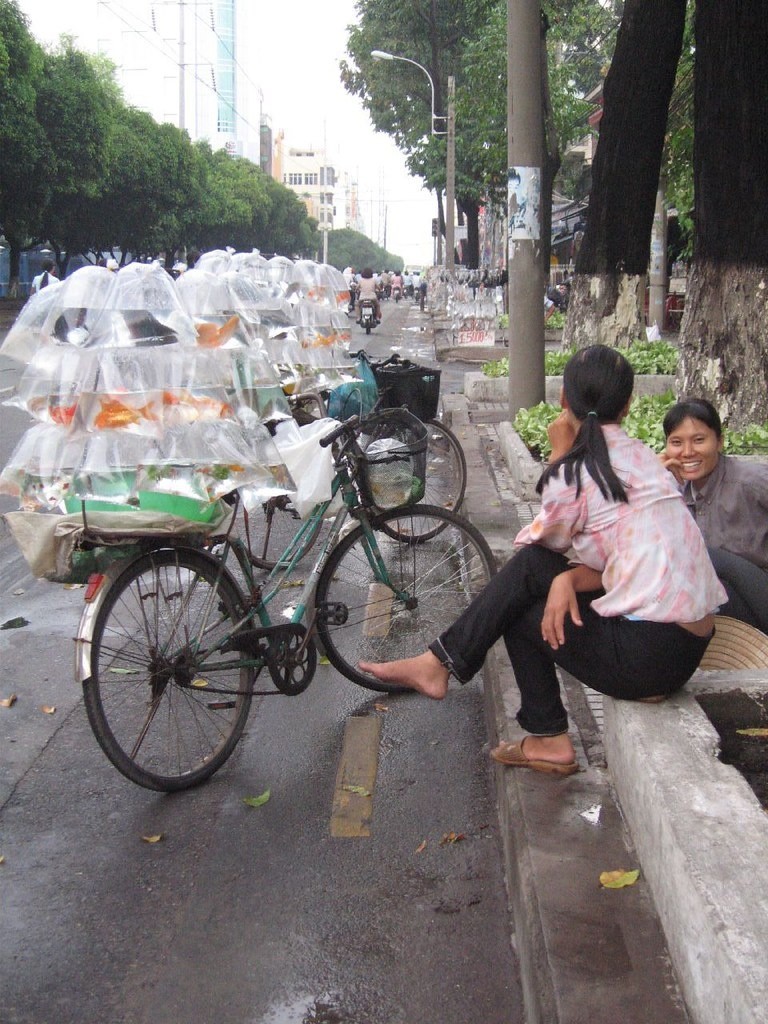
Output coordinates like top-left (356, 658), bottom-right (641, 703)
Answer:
top-left (432, 218), bottom-right (438, 237)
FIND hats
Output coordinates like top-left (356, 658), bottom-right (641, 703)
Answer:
top-left (697, 615), bottom-right (768, 673)
top-left (172, 262), bottom-right (187, 274)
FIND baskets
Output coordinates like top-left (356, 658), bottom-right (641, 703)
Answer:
top-left (377, 365), bottom-right (442, 420)
top-left (336, 407), bottom-right (429, 513)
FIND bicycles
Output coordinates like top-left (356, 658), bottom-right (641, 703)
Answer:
top-left (73, 351), bottom-right (498, 793)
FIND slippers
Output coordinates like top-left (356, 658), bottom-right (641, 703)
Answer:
top-left (490, 736), bottom-right (578, 775)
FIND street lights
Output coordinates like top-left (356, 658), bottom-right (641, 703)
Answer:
top-left (371, 50), bottom-right (456, 272)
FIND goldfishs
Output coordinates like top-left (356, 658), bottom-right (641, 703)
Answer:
top-left (27, 288), bottom-right (351, 513)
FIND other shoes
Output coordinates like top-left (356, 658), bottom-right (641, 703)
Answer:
top-left (356, 318), bottom-right (360, 324)
top-left (376, 319), bottom-right (380, 323)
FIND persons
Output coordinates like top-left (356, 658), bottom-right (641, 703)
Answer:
top-left (356, 344), bottom-right (729, 771)
top-left (555, 284), bottom-right (570, 312)
top-left (654, 397), bottom-right (768, 635)
top-left (342, 264), bottom-right (422, 321)
top-left (28, 250), bottom-right (201, 338)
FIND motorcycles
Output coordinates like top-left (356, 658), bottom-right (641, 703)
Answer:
top-left (359, 300), bottom-right (378, 335)
top-left (380, 285), bottom-right (420, 303)
